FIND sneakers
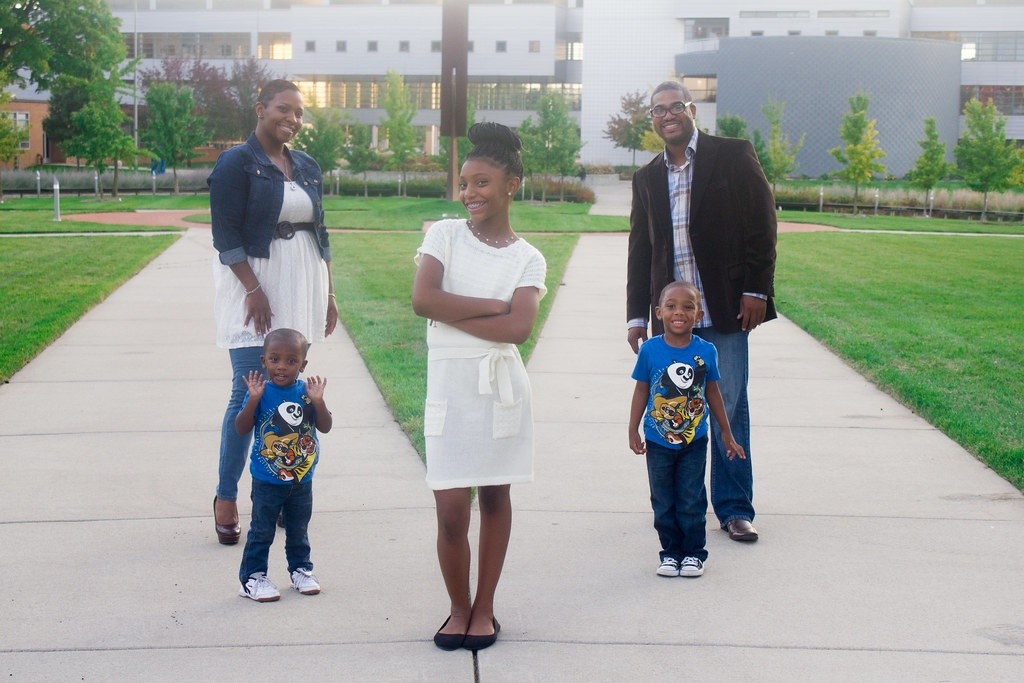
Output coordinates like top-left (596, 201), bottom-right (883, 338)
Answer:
top-left (238, 571), bottom-right (281, 602)
top-left (656, 557), bottom-right (679, 577)
top-left (679, 556), bottom-right (704, 577)
top-left (290, 566), bottom-right (320, 596)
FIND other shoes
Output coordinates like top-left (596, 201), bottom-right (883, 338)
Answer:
top-left (463, 613), bottom-right (500, 651)
top-left (434, 614), bottom-right (468, 651)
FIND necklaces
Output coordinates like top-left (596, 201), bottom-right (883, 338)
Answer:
top-left (281, 158), bottom-right (296, 191)
top-left (469, 220), bottom-right (514, 243)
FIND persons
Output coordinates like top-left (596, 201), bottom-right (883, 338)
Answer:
top-left (626, 81), bottom-right (779, 541)
top-left (206, 79), bottom-right (338, 546)
top-left (628, 281), bottom-right (746, 576)
top-left (239, 326), bottom-right (332, 603)
top-left (411, 122), bottom-right (547, 650)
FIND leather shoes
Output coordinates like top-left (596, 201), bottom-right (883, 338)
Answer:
top-left (720, 519), bottom-right (759, 542)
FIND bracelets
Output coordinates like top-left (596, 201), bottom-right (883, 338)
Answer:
top-left (244, 283), bottom-right (261, 294)
top-left (328, 293), bottom-right (336, 297)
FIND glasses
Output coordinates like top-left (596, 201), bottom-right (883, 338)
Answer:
top-left (649, 101), bottom-right (692, 119)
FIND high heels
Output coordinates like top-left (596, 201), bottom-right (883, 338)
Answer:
top-left (213, 494), bottom-right (241, 544)
top-left (276, 508), bottom-right (286, 527)
